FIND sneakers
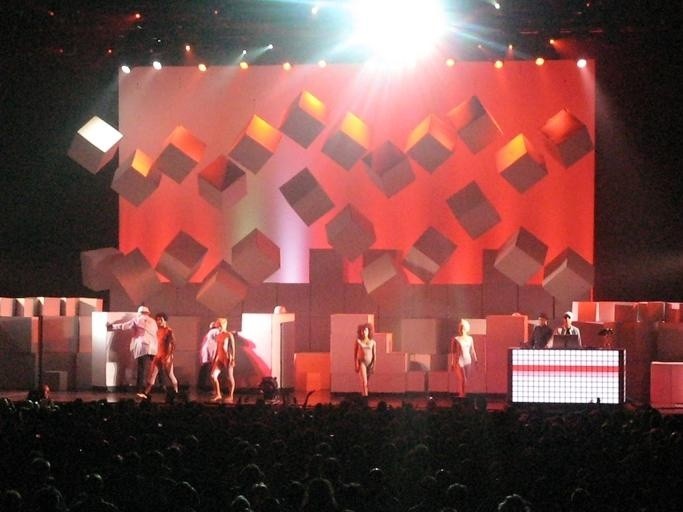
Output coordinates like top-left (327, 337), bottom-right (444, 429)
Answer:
top-left (136, 392), bottom-right (147, 399)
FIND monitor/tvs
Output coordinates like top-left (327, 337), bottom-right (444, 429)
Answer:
top-left (553, 335), bottom-right (578, 349)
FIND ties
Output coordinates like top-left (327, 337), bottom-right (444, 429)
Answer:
top-left (566, 329), bottom-right (570, 335)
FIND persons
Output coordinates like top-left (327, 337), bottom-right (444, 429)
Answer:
top-left (0, 398), bottom-right (683, 512)
top-left (197, 322), bottom-right (220, 393)
top-left (210, 317), bottom-right (236, 402)
top-left (530, 312), bottom-right (582, 349)
top-left (108, 306), bottom-right (178, 398)
top-left (354, 323), bottom-right (376, 398)
top-left (451, 319), bottom-right (478, 396)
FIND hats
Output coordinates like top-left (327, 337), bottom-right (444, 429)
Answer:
top-left (563, 312), bottom-right (574, 319)
top-left (138, 305), bottom-right (150, 312)
top-left (537, 313), bottom-right (549, 321)
